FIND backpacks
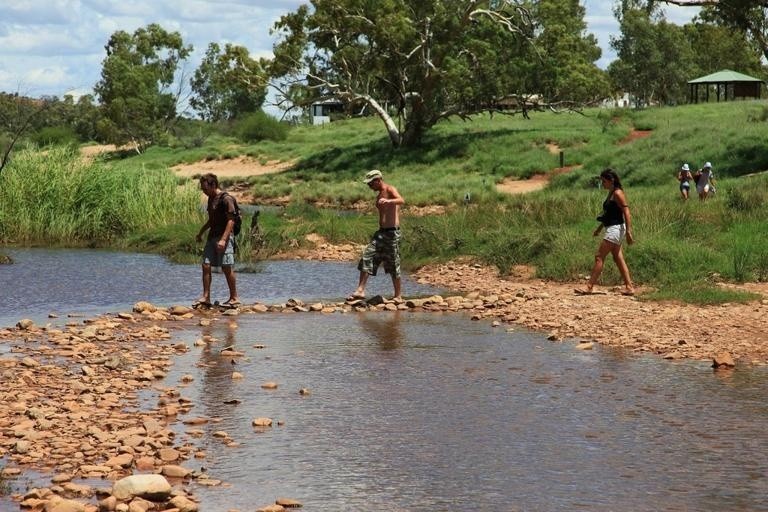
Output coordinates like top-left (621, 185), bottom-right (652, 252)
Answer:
top-left (218, 192), bottom-right (242, 236)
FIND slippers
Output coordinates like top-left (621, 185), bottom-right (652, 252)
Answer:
top-left (192, 300), bottom-right (212, 306)
top-left (220, 302), bottom-right (240, 307)
top-left (385, 298), bottom-right (405, 304)
top-left (346, 294), bottom-right (366, 301)
top-left (573, 288), bottom-right (592, 295)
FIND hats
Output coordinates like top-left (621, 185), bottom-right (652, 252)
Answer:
top-left (363, 169), bottom-right (382, 184)
top-left (704, 162), bottom-right (712, 169)
top-left (681, 163), bottom-right (689, 170)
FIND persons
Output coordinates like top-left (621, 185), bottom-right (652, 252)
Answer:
top-left (190, 173), bottom-right (242, 309)
top-left (677, 163), bottom-right (694, 201)
top-left (344, 168), bottom-right (406, 305)
top-left (694, 161), bottom-right (715, 201)
top-left (574, 168), bottom-right (634, 296)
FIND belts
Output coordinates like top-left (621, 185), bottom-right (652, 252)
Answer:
top-left (379, 226), bottom-right (400, 231)
